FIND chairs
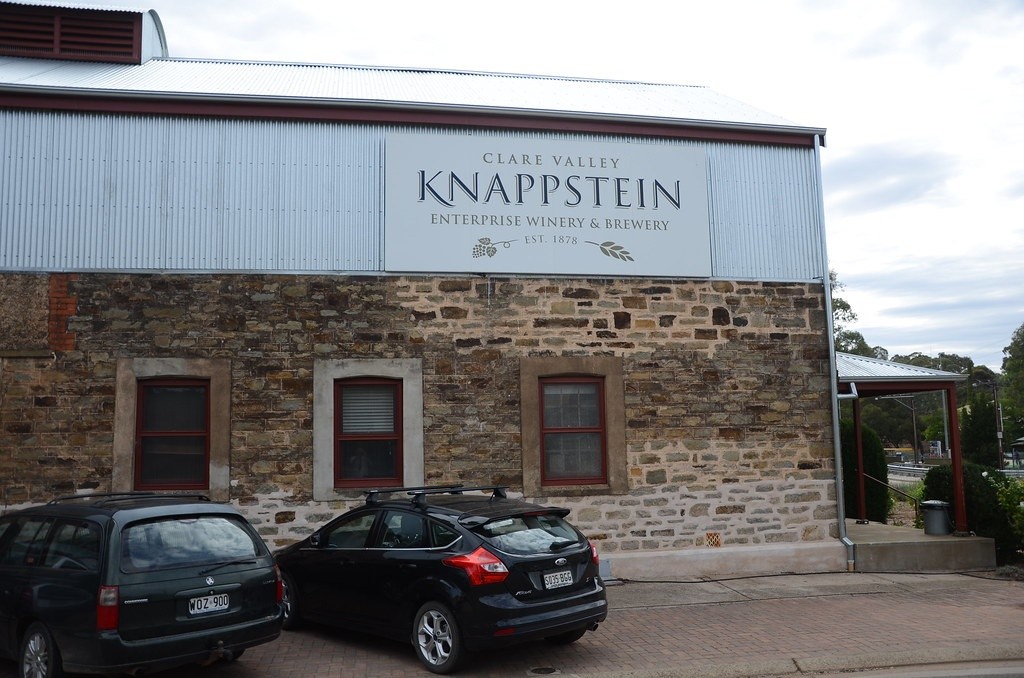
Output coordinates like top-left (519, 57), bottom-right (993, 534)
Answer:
top-left (401, 515), bottom-right (421, 539)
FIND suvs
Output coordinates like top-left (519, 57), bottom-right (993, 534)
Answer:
top-left (267, 482), bottom-right (607, 675)
top-left (0, 492), bottom-right (285, 678)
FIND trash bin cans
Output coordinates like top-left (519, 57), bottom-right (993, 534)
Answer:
top-left (895, 451), bottom-right (902, 461)
top-left (919, 500), bottom-right (952, 536)
